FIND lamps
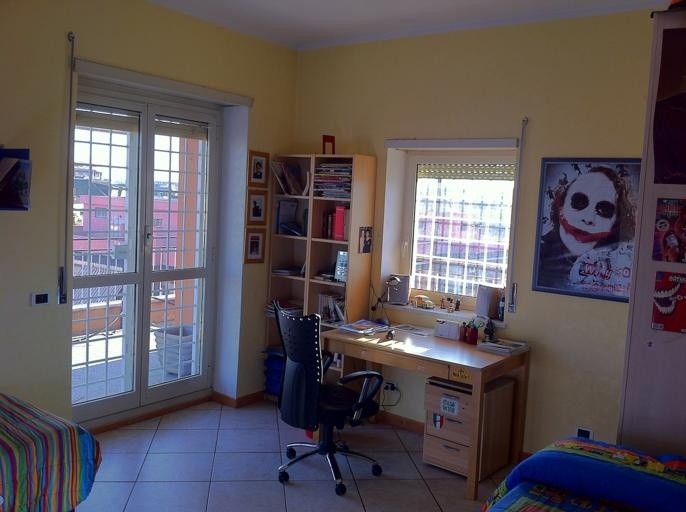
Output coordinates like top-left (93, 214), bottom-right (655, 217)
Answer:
top-left (371, 277), bottom-right (400, 326)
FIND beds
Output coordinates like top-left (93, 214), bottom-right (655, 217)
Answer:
top-left (478, 436), bottom-right (686, 512)
top-left (0, 392), bottom-right (102, 512)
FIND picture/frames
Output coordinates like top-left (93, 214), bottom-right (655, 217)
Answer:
top-left (248, 150), bottom-right (268, 188)
top-left (245, 227), bottom-right (265, 263)
top-left (247, 189), bottom-right (267, 226)
top-left (532, 155), bottom-right (642, 302)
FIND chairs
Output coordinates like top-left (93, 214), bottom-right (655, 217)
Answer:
top-left (270, 298), bottom-right (383, 495)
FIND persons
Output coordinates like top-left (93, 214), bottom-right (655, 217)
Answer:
top-left (253, 161), bottom-right (262, 179)
top-left (536, 166), bottom-right (636, 290)
top-left (363, 230), bottom-right (372, 253)
top-left (360, 231), bottom-right (365, 253)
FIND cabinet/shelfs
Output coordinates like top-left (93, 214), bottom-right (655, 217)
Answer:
top-left (423, 376), bottom-right (516, 476)
top-left (268, 155), bottom-right (377, 415)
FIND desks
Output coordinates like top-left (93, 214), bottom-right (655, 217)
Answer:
top-left (320, 319), bottom-right (530, 499)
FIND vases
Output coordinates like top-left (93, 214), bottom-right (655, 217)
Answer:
top-left (154, 323), bottom-right (193, 376)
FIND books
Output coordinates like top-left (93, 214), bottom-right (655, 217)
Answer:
top-left (265, 160), bottom-right (352, 326)
top-left (476, 338), bottom-right (529, 357)
